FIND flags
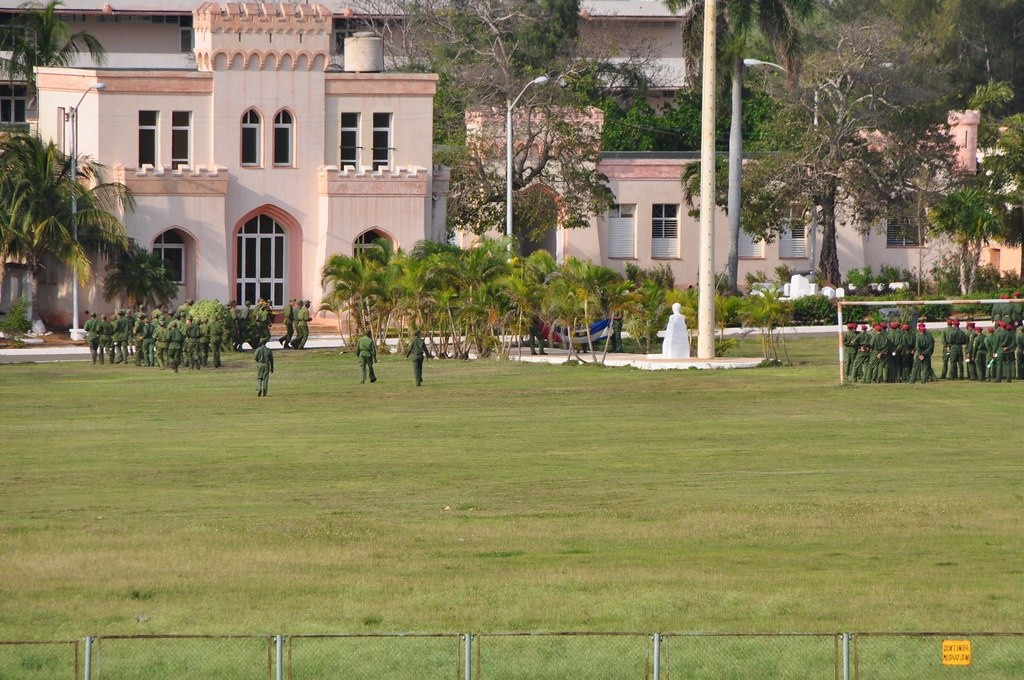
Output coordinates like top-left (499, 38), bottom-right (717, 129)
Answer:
top-left (539, 318), bottom-right (614, 343)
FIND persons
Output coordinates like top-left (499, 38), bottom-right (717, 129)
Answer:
top-left (405, 330), bottom-right (430, 386)
top-left (530, 315), bottom-right (548, 355)
top-left (609, 295), bottom-right (623, 353)
top-left (582, 343), bottom-right (587, 352)
top-left (279, 299), bottom-right (312, 350)
top-left (940, 293), bottom-right (1024, 382)
top-left (255, 338), bottom-right (273, 396)
top-left (843, 322), bottom-right (938, 385)
top-left (214, 298), bottom-right (275, 352)
top-left (84, 299), bottom-right (223, 372)
top-left (357, 329), bottom-right (378, 384)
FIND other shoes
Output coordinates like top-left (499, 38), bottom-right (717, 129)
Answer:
top-left (257, 390), bottom-right (263, 397)
top-left (94, 352), bottom-right (221, 373)
top-left (608, 350), bottom-right (616, 353)
top-left (531, 352), bottom-right (538, 355)
top-left (577, 351), bottom-right (580, 353)
top-left (261, 392), bottom-right (266, 396)
top-left (846, 376), bottom-right (1023, 385)
top-left (615, 351), bottom-right (624, 353)
top-left (370, 378), bottom-right (376, 382)
top-left (360, 380), bottom-right (364, 383)
top-left (234, 345), bottom-right (244, 352)
top-left (539, 352), bottom-right (548, 355)
top-left (583, 351), bottom-right (588, 353)
top-left (278, 339), bottom-right (304, 349)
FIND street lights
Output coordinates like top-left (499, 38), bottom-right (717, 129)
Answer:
top-left (67, 81), bottom-right (108, 343)
top-left (504, 76), bottom-right (549, 264)
top-left (741, 56), bottom-right (819, 282)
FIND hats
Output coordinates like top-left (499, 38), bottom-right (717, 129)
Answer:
top-left (847, 321), bottom-right (1024, 332)
top-left (413, 330), bottom-right (420, 335)
top-left (1000, 291), bottom-right (1021, 299)
top-left (365, 329), bottom-right (371, 334)
top-left (260, 338), bottom-right (266, 342)
top-left (90, 299), bottom-right (311, 327)
